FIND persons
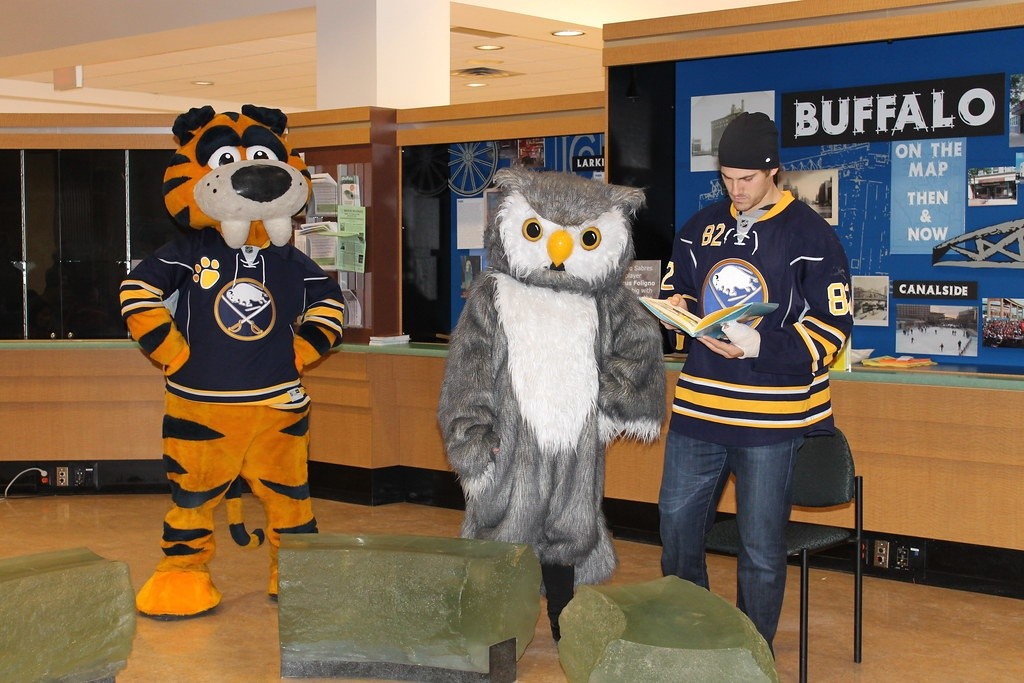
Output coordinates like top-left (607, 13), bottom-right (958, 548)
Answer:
top-left (658, 111), bottom-right (854, 661)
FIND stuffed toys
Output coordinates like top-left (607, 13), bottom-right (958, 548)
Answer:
top-left (436, 168), bottom-right (665, 641)
top-left (118, 105), bottom-right (343, 620)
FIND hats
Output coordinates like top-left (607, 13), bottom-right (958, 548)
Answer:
top-left (717, 112), bottom-right (782, 169)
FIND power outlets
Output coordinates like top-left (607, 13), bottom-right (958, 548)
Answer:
top-left (862, 538), bottom-right (926, 573)
top-left (32, 462), bottom-right (100, 493)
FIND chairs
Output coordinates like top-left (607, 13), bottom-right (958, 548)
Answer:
top-left (703, 427), bottom-right (864, 683)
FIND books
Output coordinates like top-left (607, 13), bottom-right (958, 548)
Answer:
top-left (641, 295), bottom-right (779, 336)
top-left (369, 334), bottom-right (410, 345)
